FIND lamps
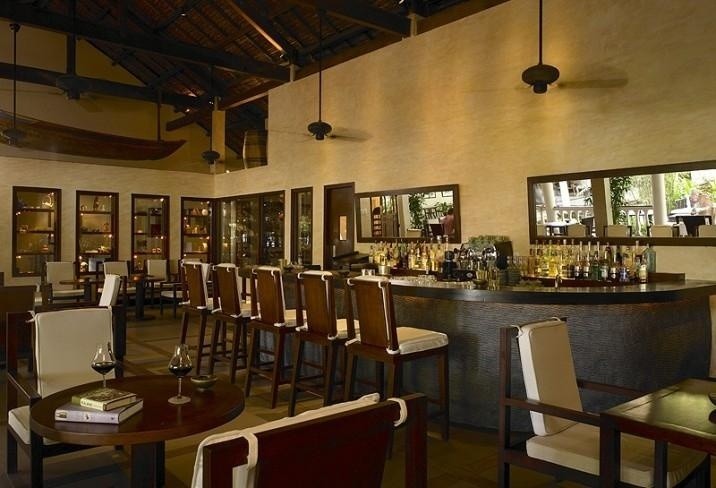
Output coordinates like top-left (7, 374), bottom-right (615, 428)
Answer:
top-left (519, 0), bottom-right (559, 93)
top-left (307, 10), bottom-right (333, 141)
top-left (2, 21), bottom-right (25, 141)
top-left (201, 66), bottom-right (219, 165)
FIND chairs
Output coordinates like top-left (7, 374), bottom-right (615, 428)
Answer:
top-left (497, 317), bottom-right (711, 488)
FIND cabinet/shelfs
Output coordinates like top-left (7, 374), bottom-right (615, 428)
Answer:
top-left (11, 184), bottom-right (214, 279)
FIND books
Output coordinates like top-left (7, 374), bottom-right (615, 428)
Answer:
top-left (54, 398), bottom-right (144, 425)
top-left (70, 387), bottom-right (138, 412)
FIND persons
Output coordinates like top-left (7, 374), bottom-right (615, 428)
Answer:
top-left (439, 207), bottom-right (455, 236)
top-left (693, 193), bottom-right (715, 209)
top-left (686, 189), bottom-right (700, 207)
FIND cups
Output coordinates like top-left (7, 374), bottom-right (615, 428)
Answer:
top-left (453, 243), bottom-right (498, 274)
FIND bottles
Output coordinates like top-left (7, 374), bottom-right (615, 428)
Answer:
top-left (507, 239), bottom-right (660, 281)
top-left (366, 238), bottom-right (443, 272)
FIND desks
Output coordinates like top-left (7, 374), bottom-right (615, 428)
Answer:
top-left (598, 376), bottom-right (716, 488)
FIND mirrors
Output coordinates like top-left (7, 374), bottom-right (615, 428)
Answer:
top-left (353, 182), bottom-right (461, 245)
top-left (526, 158), bottom-right (716, 247)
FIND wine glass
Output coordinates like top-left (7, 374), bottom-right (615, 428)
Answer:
top-left (89, 340), bottom-right (116, 385)
top-left (167, 343), bottom-right (191, 403)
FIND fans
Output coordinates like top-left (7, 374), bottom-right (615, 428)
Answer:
top-left (0, 75), bottom-right (147, 113)
